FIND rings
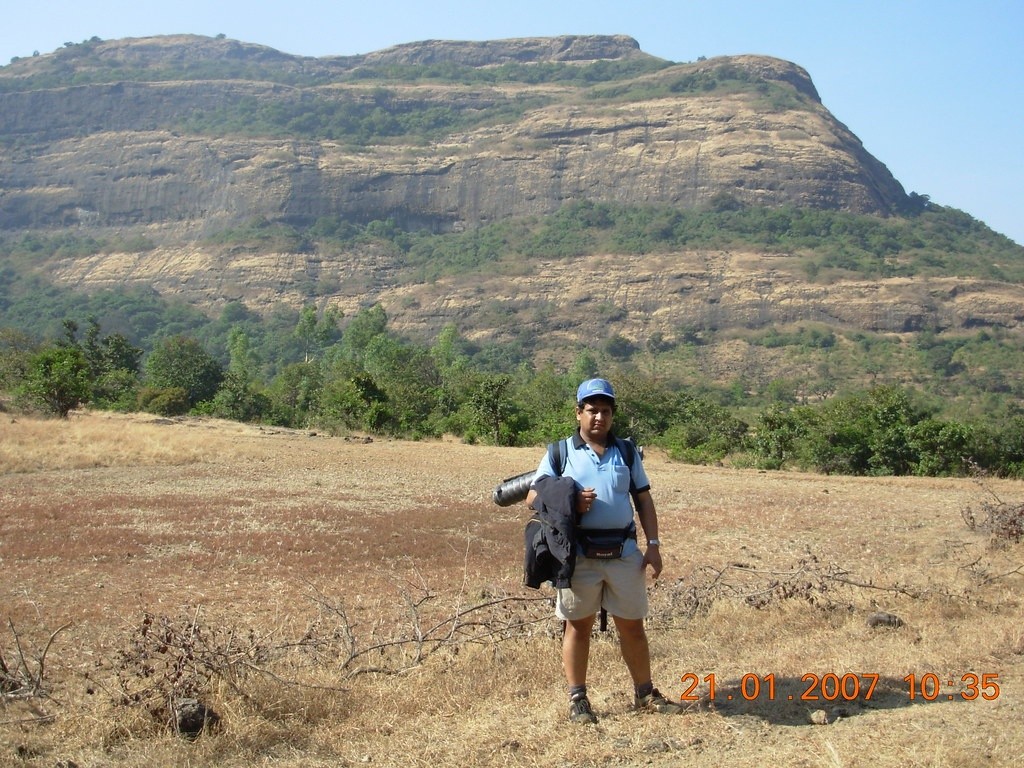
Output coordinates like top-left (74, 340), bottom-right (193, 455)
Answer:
top-left (588, 503), bottom-right (590, 507)
top-left (586, 507), bottom-right (590, 512)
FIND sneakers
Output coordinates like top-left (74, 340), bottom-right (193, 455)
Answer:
top-left (635, 689), bottom-right (684, 715)
top-left (567, 693), bottom-right (597, 725)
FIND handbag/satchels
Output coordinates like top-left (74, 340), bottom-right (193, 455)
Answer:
top-left (573, 524), bottom-right (636, 560)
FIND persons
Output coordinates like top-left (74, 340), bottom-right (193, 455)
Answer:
top-left (525, 377), bottom-right (684, 726)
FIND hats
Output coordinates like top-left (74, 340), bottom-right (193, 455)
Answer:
top-left (576, 378), bottom-right (615, 404)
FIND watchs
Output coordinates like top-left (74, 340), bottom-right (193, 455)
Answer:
top-left (646, 539), bottom-right (660, 547)
